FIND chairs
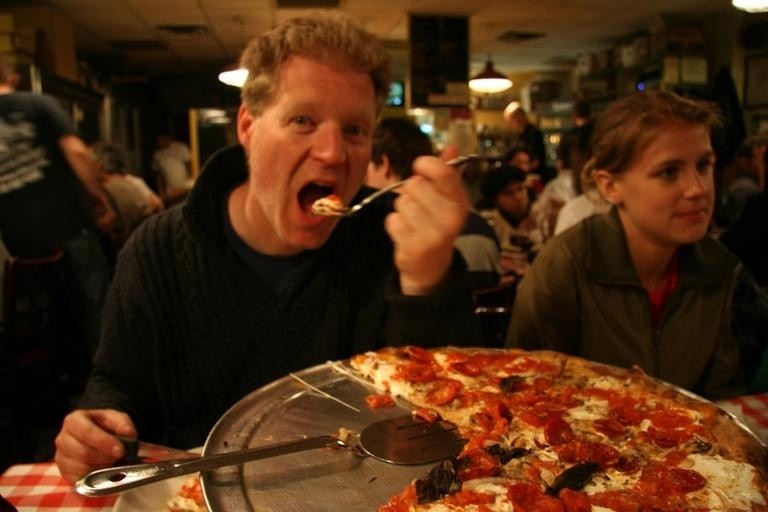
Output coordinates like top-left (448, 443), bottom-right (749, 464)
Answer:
top-left (471, 283), bottom-right (516, 331)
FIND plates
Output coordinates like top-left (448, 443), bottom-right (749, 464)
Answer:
top-left (197, 352), bottom-right (765, 512)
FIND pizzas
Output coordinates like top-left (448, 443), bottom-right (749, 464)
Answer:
top-left (164, 472), bottom-right (209, 512)
top-left (350, 344), bottom-right (768, 512)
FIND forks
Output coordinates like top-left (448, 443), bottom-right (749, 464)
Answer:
top-left (310, 149), bottom-right (492, 217)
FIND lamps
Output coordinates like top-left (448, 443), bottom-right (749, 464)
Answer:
top-left (467, 49), bottom-right (514, 94)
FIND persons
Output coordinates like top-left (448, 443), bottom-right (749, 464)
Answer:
top-left (702, 100), bottom-right (768, 286)
top-left (503, 86), bottom-right (768, 399)
top-left (152, 128), bottom-right (195, 207)
top-left (93, 143), bottom-right (163, 222)
top-left (50, 10), bottom-right (487, 488)
top-left (366, 101), bottom-right (613, 276)
top-left (0, 56), bottom-right (120, 377)
